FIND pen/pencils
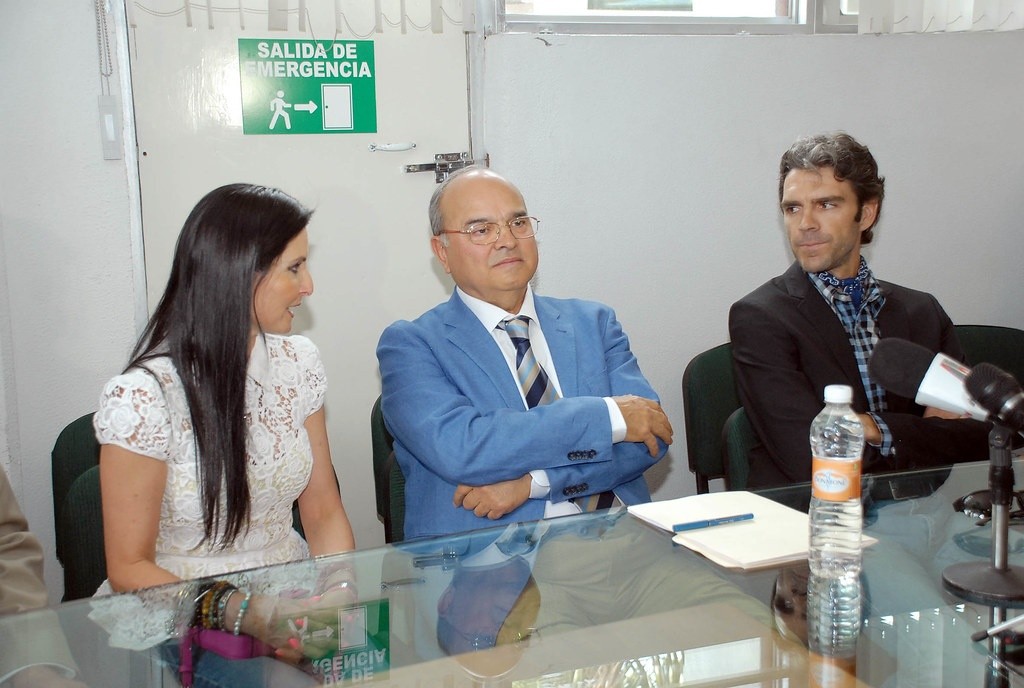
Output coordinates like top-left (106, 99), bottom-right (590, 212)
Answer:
top-left (673, 512), bottom-right (755, 533)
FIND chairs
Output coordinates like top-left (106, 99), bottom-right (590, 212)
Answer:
top-left (682, 342), bottom-right (789, 492)
top-left (51, 411), bottom-right (108, 603)
top-left (952, 325), bottom-right (1024, 463)
top-left (370, 393), bottom-right (406, 545)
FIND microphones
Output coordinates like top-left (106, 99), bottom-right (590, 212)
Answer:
top-left (867, 337), bottom-right (999, 426)
top-left (962, 361), bottom-right (1024, 437)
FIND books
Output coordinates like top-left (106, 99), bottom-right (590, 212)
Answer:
top-left (627, 490), bottom-right (878, 569)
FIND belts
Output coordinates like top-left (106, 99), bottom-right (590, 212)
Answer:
top-left (861, 470), bottom-right (952, 502)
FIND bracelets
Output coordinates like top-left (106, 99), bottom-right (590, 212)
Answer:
top-left (315, 565), bottom-right (356, 604)
top-left (168, 577), bottom-right (250, 635)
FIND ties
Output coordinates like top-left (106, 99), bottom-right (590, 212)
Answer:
top-left (495, 520), bottom-right (539, 558)
top-left (497, 317), bottom-right (621, 512)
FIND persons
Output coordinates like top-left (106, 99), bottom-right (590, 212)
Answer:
top-left (376, 164), bottom-right (806, 657)
top-left (0, 463), bottom-right (89, 688)
top-left (729, 131), bottom-right (1024, 688)
top-left (86, 183), bottom-right (391, 688)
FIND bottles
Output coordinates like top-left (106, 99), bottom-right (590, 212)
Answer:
top-left (806, 384), bottom-right (865, 577)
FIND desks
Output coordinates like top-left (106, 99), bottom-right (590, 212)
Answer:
top-left (0, 456), bottom-right (1024, 687)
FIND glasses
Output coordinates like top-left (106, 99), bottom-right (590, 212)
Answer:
top-left (444, 216), bottom-right (540, 245)
top-left (952, 488), bottom-right (1024, 527)
top-left (953, 524), bottom-right (1024, 561)
top-left (446, 619), bottom-right (545, 650)
